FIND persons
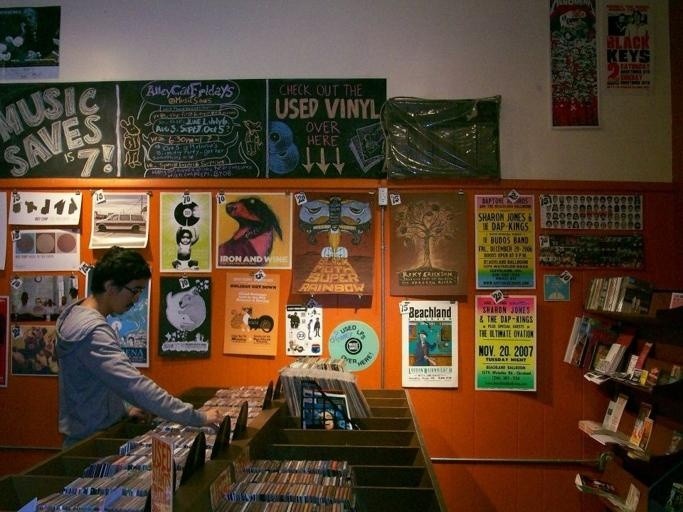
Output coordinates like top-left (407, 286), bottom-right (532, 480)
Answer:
top-left (55, 246), bottom-right (222, 452)
top-left (172, 226), bottom-right (199, 271)
top-left (17, 286), bottom-right (78, 314)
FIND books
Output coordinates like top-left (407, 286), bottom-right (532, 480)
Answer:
top-left (131, 408), bottom-right (238, 450)
top-left (204, 383), bottom-right (274, 414)
top-left (281, 356), bottom-right (369, 432)
top-left (209, 452), bottom-right (358, 512)
top-left (577, 391), bottom-right (682, 462)
top-left (567, 319), bottom-right (682, 391)
top-left (26, 449), bottom-right (194, 511)
top-left (586, 272), bottom-right (683, 325)
top-left (569, 472), bottom-right (682, 511)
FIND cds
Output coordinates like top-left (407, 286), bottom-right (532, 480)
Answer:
top-left (269, 143), bottom-right (300, 175)
top-left (268, 120), bottom-right (293, 155)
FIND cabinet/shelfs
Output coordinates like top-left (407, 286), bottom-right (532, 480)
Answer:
top-left (581, 289), bottom-right (683, 512)
top-left (0, 387), bottom-right (287, 512)
top-left (182, 389), bottom-right (449, 511)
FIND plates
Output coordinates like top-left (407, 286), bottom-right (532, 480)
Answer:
top-left (15, 233), bottom-right (77, 253)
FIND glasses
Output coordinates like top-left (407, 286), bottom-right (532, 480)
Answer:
top-left (125, 286), bottom-right (144, 295)
